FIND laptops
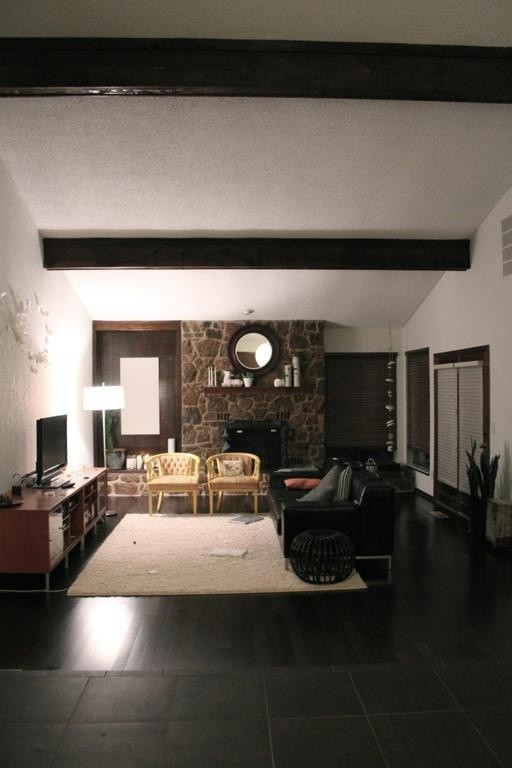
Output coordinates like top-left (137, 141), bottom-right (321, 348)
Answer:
top-left (230, 512), bottom-right (263, 525)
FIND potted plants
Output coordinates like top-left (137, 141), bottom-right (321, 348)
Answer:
top-left (241, 372), bottom-right (254, 387)
top-left (97, 410), bottom-right (128, 470)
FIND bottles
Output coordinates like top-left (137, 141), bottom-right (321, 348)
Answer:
top-left (126, 455), bottom-right (142, 470)
top-left (285, 353), bottom-right (301, 387)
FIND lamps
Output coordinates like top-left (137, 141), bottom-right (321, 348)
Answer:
top-left (82, 382), bottom-right (126, 518)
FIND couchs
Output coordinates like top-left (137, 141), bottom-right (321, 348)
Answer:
top-left (268, 458), bottom-right (395, 558)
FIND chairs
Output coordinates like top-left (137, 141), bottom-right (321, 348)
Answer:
top-left (205, 453), bottom-right (261, 515)
top-left (145, 453), bottom-right (200, 515)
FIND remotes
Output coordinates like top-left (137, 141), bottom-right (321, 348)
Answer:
top-left (61, 481), bottom-right (75, 488)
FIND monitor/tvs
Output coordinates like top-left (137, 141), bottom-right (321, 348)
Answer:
top-left (36, 415), bottom-right (69, 488)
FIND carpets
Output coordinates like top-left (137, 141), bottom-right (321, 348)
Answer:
top-left (66, 512), bottom-right (367, 597)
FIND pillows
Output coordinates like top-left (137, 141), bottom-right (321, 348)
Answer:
top-left (283, 478), bottom-right (320, 489)
top-left (335, 465), bottom-right (353, 500)
top-left (221, 460), bottom-right (244, 477)
top-left (295, 464), bottom-right (341, 502)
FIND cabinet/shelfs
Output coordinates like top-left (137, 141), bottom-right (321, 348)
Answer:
top-left (0, 467), bottom-right (108, 589)
top-left (484, 497), bottom-right (512, 545)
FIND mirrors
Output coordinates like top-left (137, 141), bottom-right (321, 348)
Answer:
top-left (228, 324), bottom-right (280, 377)
